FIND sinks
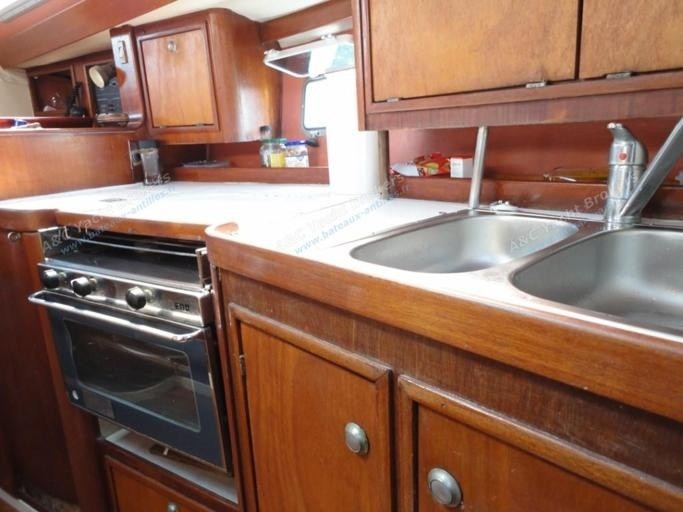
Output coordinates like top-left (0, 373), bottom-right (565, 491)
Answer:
top-left (349, 209), bottom-right (579, 275)
top-left (508, 224), bottom-right (683, 338)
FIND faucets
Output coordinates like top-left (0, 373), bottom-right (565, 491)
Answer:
top-left (601, 121), bottom-right (683, 225)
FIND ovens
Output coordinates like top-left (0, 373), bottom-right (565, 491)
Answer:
top-left (26, 225), bottom-right (235, 482)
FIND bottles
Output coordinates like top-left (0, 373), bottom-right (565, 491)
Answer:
top-left (137, 139), bottom-right (164, 186)
top-left (257, 137), bottom-right (287, 168)
top-left (39, 88), bottom-right (64, 112)
top-left (283, 139), bottom-right (309, 169)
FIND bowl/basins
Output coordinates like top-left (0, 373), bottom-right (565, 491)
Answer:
top-left (93, 110), bottom-right (128, 129)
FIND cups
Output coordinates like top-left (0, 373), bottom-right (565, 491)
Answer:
top-left (0, 118), bottom-right (13, 129)
top-left (13, 120), bottom-right (26, 127)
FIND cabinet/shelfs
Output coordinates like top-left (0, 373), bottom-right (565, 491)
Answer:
top-left (136, 10), bottom-right (277, 145)
top-left (0, 227), bottom-right (78, 507)
top-left (359, 0), bottom-right (683, 126)
top-left (209, 237), bottom-right (680, 511)
top-left (101, 447), bottom-right (237, 512)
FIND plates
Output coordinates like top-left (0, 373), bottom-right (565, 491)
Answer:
top-left (541, 164), bottom-right (607, 184)
top-left (182, 160), bottom-right (230, 169)
